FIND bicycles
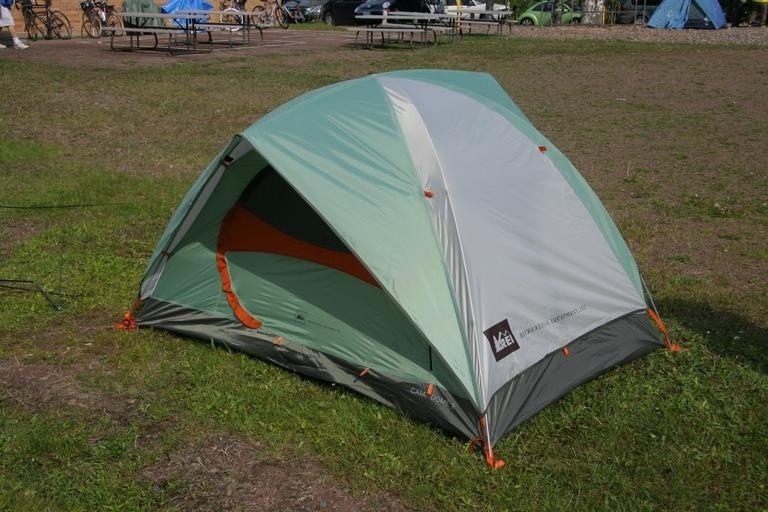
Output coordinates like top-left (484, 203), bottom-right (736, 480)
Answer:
top-left (14, 0), bottom-right (125, 40)
top-left (218, 1), bottom-right (306, 33)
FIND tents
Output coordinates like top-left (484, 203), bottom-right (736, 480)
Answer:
top-left (645, 0), bottom-right (729, 31)
top-left (116, 68), bottom-right (682, 471)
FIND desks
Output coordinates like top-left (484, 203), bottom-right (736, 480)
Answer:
top-left (120, 9), bottom-right (258, 53)
top-left (355, 8), bottom-right (513, 48)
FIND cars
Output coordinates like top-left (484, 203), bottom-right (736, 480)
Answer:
top-left (516, 0), bottom-right (583, 26)
top-left (280, 1), bottom-right (514, 26)
top-left (614, 0), bottom-right (660, 27)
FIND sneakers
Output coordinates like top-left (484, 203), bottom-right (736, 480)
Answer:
top-left (13, 41), bottom-right (29, 51)
top-left (1, 43), bottom-right (7, 49)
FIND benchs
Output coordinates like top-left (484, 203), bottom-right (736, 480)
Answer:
top-left (354, 19), bottom-right (518, 50)
top-left (98, 22), bottom-right (263, 55)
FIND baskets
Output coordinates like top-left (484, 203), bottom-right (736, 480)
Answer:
top-left (81, 2), bottom-right (93, 10)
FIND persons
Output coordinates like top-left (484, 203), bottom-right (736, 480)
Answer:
top-left (0, 0), bottom-right (30, 51)
